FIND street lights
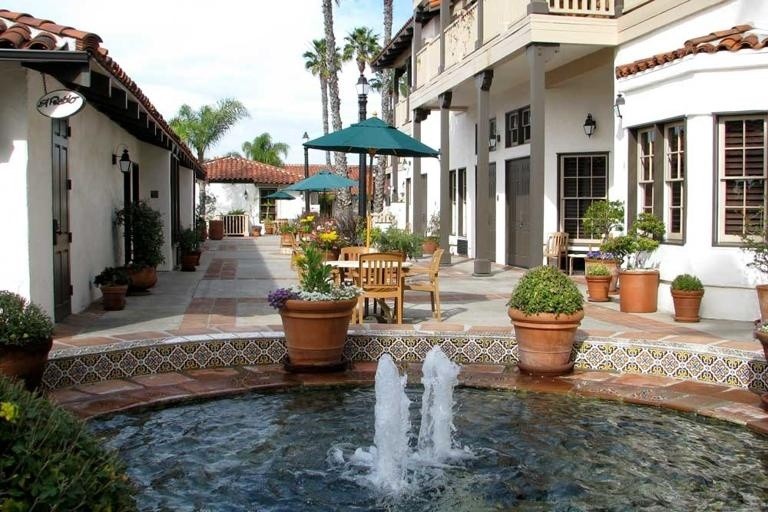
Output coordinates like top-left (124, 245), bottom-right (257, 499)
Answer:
top-left (302, 131), bottom-right (310, 212)
top-left (355, 75), bottom-right (370, 218)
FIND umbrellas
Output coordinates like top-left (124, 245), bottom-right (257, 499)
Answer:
top-left (260, 191), bottom-right (296, 200)
top-left (280, 167), bottom-right (359, 192)
top-left (301, 112), bottom-right (442, 253)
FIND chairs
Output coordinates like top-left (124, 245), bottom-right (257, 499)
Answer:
top-left (403, 248), bottom-right (444, 321)
top-left (355, 253), bottom-right (403, 324)
top-left (543, 234), bottom-right (561, 271)
top-left (338, 246), bottom-right (381, 315)
top-left (560, 232), bottom-right (569, 275)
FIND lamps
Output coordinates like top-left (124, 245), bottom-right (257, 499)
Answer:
top-left (584, 113), bottom-right (596, 138)
top-left (489, 134), bottom-right (496, 149)
top-left (119, 148), bottom-right (131, 173)
top-left (244, 190), bottom-right (248, 199)
top-left (615, 93), bottom-right (625, 107)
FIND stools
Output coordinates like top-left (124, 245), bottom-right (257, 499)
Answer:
top-left (569, 251), bottom-right (588, 277)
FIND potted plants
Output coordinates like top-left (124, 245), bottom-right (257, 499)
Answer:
top-left (737, 201), bottom-right (766, 323)
top-left (508, 265), bottom-right (584, 375)
top-left (583, 201), bottom-right (624, 294)
top-left (755, 326), bottom-right (768, 405)
top-left (95, 267), bottom-right (129, 310)
top-left (265, 217), bottom-right (273, 234)
top-left (196, 214), bottom-right (208, 241)
top-left (114, 200), bottom-right (167, 296)
top-left (267, 227), bottom-right (364, 367)
top-left (670, 274), bottom-right (704, 322)
top-left (249, 205), bottom-right (261, 237)
top-left (378, 222), bottom-right (422, 262)
top-left (423, 213), bottom-right (442, 254)
top-left (292, 250), bottom-right (323, 283)
top-left (617, 213), bottom-right (666, 314)
top-left (220, 209), bottom-right (249, 239)
top-left (190, 226), bottom-right (201, 267)
top-left (313, 220), bottom-right (343, 260)
top-left (172, 228), bottom-right (197, 272)
top-left (278, 226), bottom-right (296, 246)
top-left (1, 291), bottom-right (55, 388)
top-left (584, 264), bottom-right (614, 302)
